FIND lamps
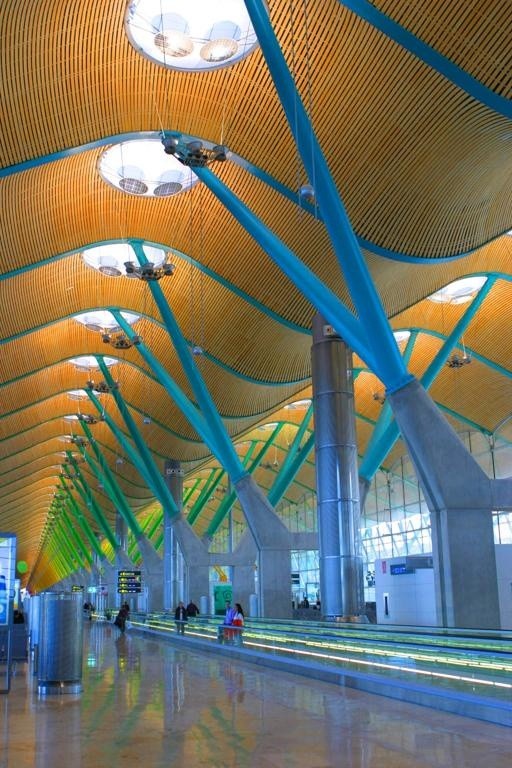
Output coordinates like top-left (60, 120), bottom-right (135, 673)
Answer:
top-left (77, 238), bottom-right (171, 282)
top-left (118, 3), bottom-right (260, 78)
top-left (93, 135), bottom-right (201, 202)
top-left (427, 276), bottom-right (488, 307)
top-left (69, 350), bottom-right (116, 371)
top-left (70, 307), bottom-right (144, 336)
top-left (71, 385), bottom-right (106, 403)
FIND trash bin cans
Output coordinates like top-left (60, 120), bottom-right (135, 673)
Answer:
top-left (24, 591), bottom-right (84, 694)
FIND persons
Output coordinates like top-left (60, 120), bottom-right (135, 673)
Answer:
top-left (303, 597), bottom-right (309, 608)
top-left (83, 602), bottom-right (89, 612)
top-left (117, 605), bottom-right (129, 633)
top-left (175, 600), bottom-right (187, 635)
top-left (121, 601), bottom-right (130, 612)
top-left (216, 600), bottom-right (235, 642)
top-left (185, 600), bottom-right (200, 617)
top-left (13, 607), bottom-right (24, 624)
top-left (224, 603), bottom-right (244, 638)
top-left (316, 598), bottom-right (320, 610)
top-left (88, 602), bottom-right (94, 613)
